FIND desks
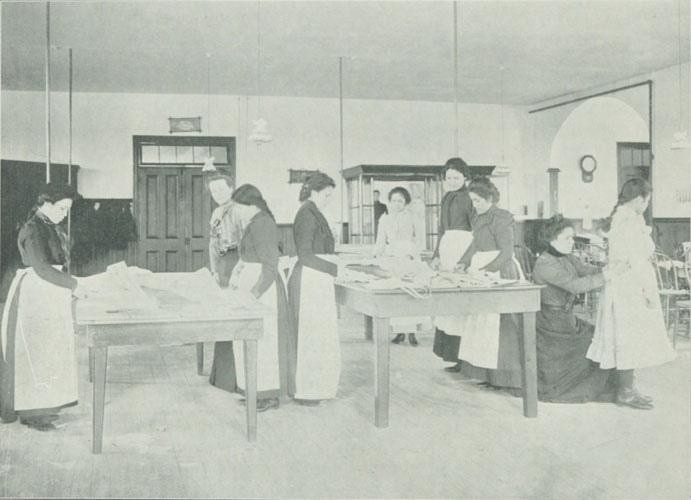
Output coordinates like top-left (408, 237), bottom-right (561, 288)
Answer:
top-left (75, 283), bottom-right (273, 454)
top-left (335, 273), bottom-right (547, 429)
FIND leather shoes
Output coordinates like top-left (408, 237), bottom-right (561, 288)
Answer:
top-left (482, 386), bottom-right (502, 392)
top-left (393, 333), bottom-right (406, 344)
top-left (255, 400), bottom-right (280, 412)
top-left (241, 397), bottom-right (260, 406)
top-left (477, 381), bottom-right (492, 386)
top-left (446, 364), bottom-right (462, 373)
top-left (21, 414), bottom-right (59, 424)
top-left (409, 333), bottom-right (418, 345)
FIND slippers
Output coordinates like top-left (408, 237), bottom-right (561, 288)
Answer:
top-left (28, 418), bottom-right (56, 432)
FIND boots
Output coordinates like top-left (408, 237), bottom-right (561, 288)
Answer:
top-left (616, 371), bottom-right (654, 412)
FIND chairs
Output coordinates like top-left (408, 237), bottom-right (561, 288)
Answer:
top-left (649, 250), bottom-right (689, 350)
top-left (514, 244), bottom-right (536, 281)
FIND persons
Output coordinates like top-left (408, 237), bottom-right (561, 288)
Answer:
top-left (204, 174), bottom-right (246, 396)
top-left (227, 182), bottom-right (288, 414)
top-left (284, 172), bottom-right (353, 405)
top-left (0, 179), bottom-right (89, 434)
top-left (452, 174), bottom-right (526, 393)
top-left (508, 213), bottom-right (618, 406)
top-left (429, 155), bottom-right (477, 373)
top-left (584, 174), bottom-right (678, 410)
top-left (373, 185), bottom-right (420, 348)
top-left (374, 189), bottom-right (388, 243)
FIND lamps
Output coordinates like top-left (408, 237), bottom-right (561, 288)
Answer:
top-left (202, 52), bottom-right (215, 173)
top-left (249, 1), bottom-right (272, 147)
top-left (669, 0), bottom-right (691, 151)
